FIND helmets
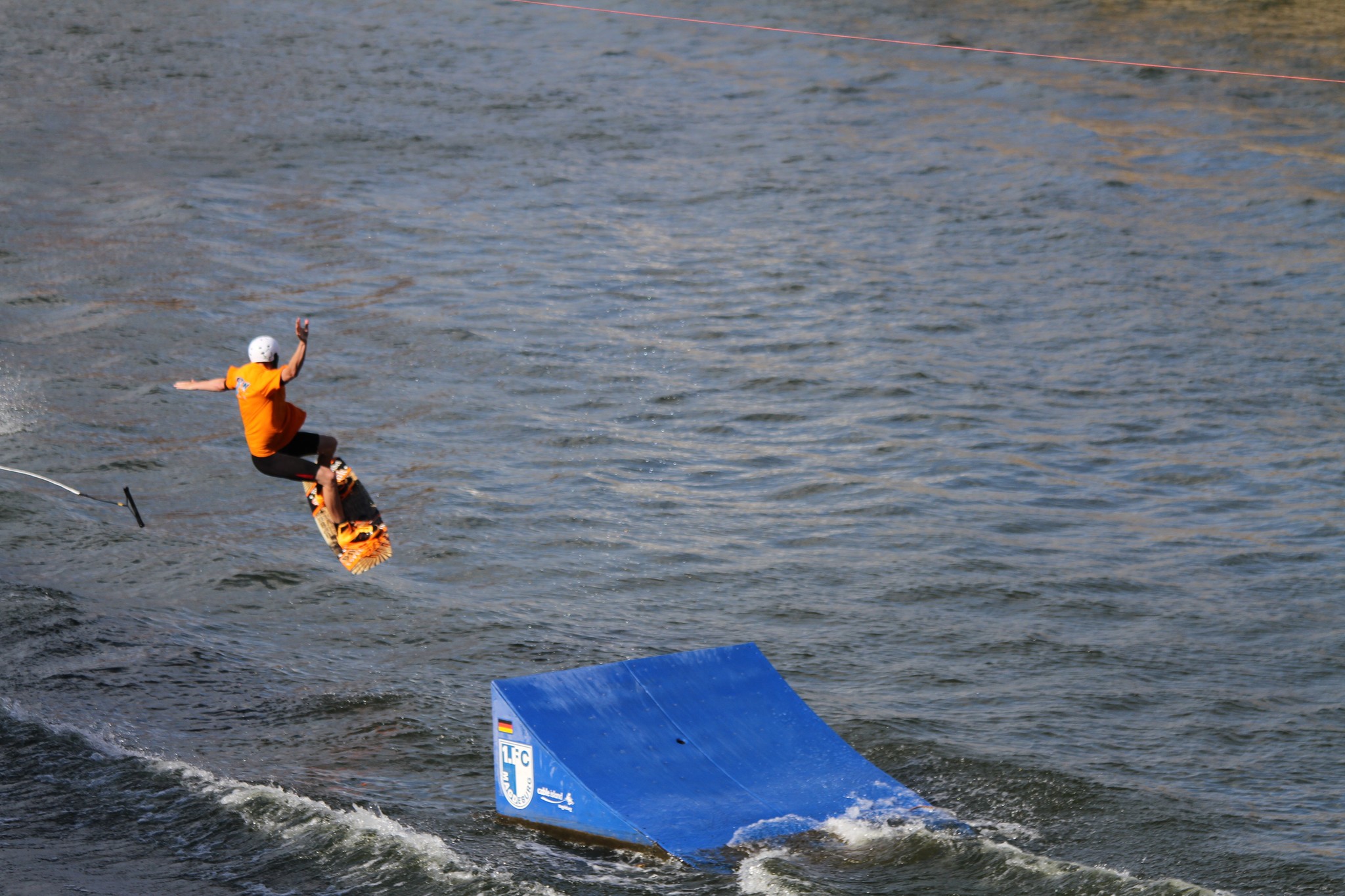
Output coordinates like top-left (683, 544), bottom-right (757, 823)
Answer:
top-left (247, 335), bottom-right (280, 364)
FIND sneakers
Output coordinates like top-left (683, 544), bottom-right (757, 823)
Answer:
top-left (335, 521), bottom-right (382, 549)
top-left (313, 477), bottom-right (353, 507)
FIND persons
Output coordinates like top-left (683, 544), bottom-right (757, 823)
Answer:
top-left (174, 317), bottom-right (380, 548)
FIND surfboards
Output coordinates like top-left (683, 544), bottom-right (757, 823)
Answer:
top-left (296, 452), bottom-right (395, 574)
